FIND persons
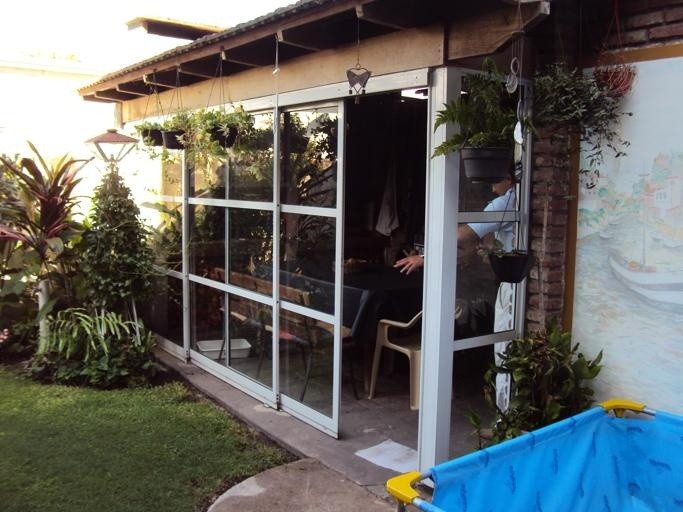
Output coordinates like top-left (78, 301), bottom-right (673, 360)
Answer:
top-left (352, 225), bottom-right (422, 370)
top-left (393, 164), bottom-right (519, 424)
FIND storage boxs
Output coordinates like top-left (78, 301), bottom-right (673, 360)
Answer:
top-left (197, 338), bottom-right (251, 361)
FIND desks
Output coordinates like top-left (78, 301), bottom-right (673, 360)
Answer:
top-left (321, 264), bottom-right (421, 379)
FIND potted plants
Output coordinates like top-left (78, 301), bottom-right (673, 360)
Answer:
top-left (159, 120), bottom-right (187, 167)
top-left (429, 57), bottom-right (539, 184)
top-left (476, 241), bottom-right (538, 286)
top-left (133, 122), bottom-right (161, 160)
top-left (175, 105), bottom-right (259, 181)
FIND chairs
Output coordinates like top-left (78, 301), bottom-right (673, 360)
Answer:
top-left (367, 310), bottom-right (421, 410)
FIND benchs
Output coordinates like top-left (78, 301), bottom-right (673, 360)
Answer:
top-left (214, 267), bottom-right (361, 403)
